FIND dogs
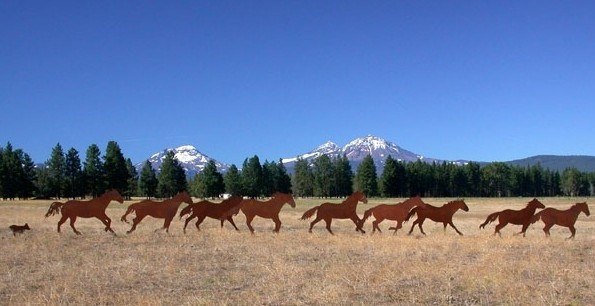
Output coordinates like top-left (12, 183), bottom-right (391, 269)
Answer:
top-left (9, 223), bottom-right (31, 236)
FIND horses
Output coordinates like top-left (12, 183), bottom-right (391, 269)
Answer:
top-left (301, 190), bottom-right (368, 234)
top-left (356, 195), bottom-right (424, 235)
top-left (531, 201), bottom-right (590, 238)
top-left (180, 194), bottom-right (242, 234)
top-left (121, 191), bottom-right (192, 234)
top-left (240, 191), bottom-right (296, 233)
top-left (45, 189), bottom-right (124, 234)
top-left (480, 197), bottom-right (545, 238)
top-left (403, 198), bottom-right (469, 237)
